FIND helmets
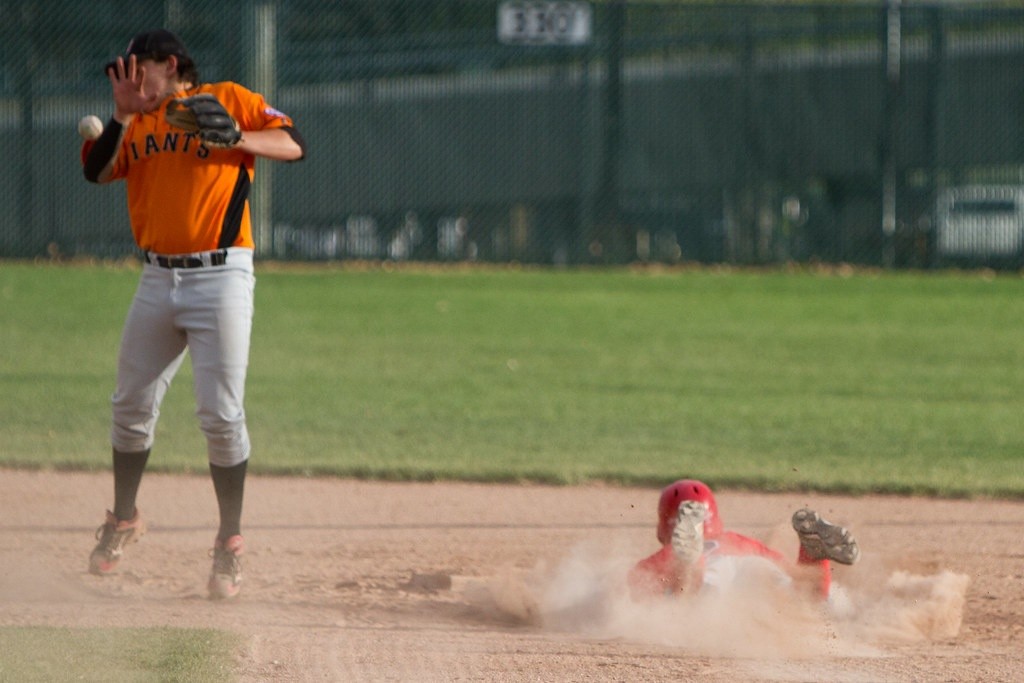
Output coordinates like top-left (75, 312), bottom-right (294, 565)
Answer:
top-left (656, 479), bottom-right (721, 544)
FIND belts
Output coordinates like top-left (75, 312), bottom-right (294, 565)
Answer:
top-left (144, 251), bottom-right (224, 268)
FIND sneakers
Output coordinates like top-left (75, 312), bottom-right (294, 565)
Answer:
top-left (208, 536), bottom-right (245, 601)
top-left (89, 507), bottom-right (146, 575)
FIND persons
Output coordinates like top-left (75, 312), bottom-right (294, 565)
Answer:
top-left (631, 479), bottom-right (860, 600)
top-left (81, 30), bottom-right (303, 598)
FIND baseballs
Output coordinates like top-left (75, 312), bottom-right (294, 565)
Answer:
top-left (76, 114), bottom-right (104, 140)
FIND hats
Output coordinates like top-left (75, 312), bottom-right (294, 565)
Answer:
top-left (105, 29), bottom-right (191, 79)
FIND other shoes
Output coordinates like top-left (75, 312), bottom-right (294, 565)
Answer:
top-left (671, 500), bottom-right (704, 563)
top-left (792, 508), bottom-right (861, 566)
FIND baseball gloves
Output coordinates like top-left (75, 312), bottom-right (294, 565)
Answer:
top-left (164, 91), bottom-right (246, 152)
